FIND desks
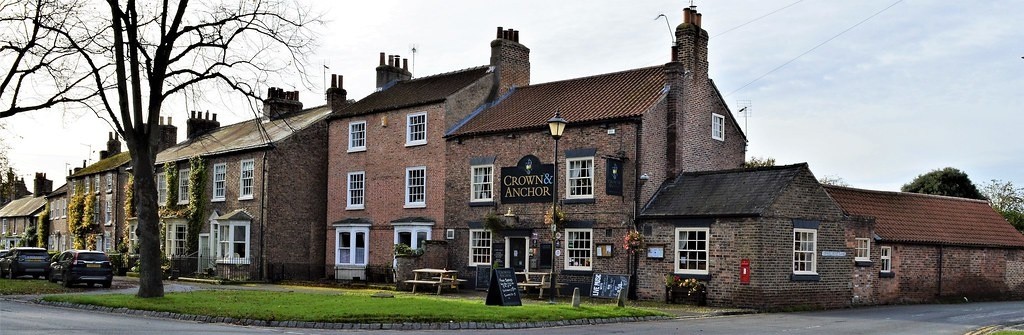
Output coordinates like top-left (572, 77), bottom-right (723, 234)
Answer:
top-left (515, 272), bottom-right (560, 298)
top-left (412, 268), bottom-right (459, 296)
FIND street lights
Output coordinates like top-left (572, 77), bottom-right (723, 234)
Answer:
top-left (654, 14), bottom-right (674, 46)
top-left (739, 107), bottom-right (747, 139)
top-left (547, 109), bottom-right (568, 304)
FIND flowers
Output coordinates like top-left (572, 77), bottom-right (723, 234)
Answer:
top-left (663, 274), bottom-right (708, 298)
top-left (621, 231), bottom-right (645, 258)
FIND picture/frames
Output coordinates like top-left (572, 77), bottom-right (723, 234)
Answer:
top-left (590, 273), bottom-right (631, 299)
top-left (646, 244), bottom-right (665, 258)
top-left (596, 243), bottom-right (614, 257)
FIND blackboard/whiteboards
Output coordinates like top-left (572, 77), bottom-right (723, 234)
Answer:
top-left (476, 266), bottom-right (492, 290)
top-left (495, 268), bottom-right (522, 305)
top-left (492, 244), bottom-right (505, 269)
top-left (591, 274), bottom-right (631, 300)
top-left (539, 244), bottom-right (554, 269)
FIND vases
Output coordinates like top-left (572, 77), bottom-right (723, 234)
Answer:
top-left (666, 285), bottom-right (706, 305)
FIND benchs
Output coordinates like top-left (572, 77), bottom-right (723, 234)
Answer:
top-left (431, 277), bottom-right (468, 282)
top-left (526, 280), bottom-right (568, 288)
top-left (518, 283), bottom-right (545, 287)
top-left (405, 279), bottom-right (442, 285)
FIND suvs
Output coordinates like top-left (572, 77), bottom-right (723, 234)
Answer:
top-left (0, 247), bottom-right (51, 279)
top-left (48, 250), bottom-right (113, 288)
